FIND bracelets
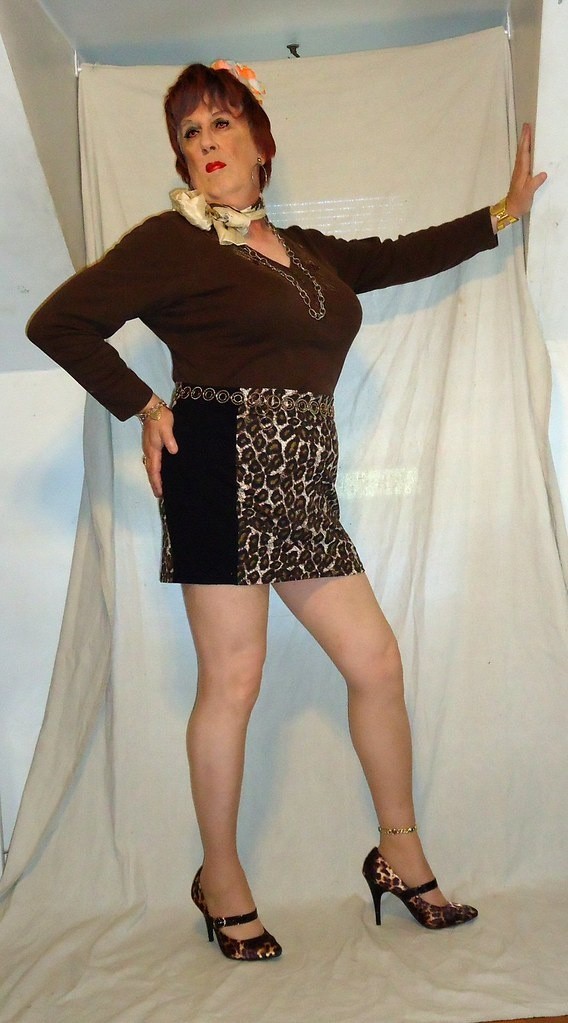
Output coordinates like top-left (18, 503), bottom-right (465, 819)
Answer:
top-left (491, 197), bottom-right (519, 231)
top-left (137, 398), bottom-right (167, 421)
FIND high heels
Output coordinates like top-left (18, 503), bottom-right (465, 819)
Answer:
top-left (362, 846), bottom-right (479, 930)
top-left (190, 865), bottom-right (283, 961)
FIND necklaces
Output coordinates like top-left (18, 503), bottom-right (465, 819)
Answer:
top-left (239, 215), bottom-right (327, 320)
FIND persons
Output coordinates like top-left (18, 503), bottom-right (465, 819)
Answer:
top-left (24, 61), bottom-right (547, 959)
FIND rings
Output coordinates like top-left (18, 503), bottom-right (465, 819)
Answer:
top-left (143, 457), bottom-right (147, 464)
